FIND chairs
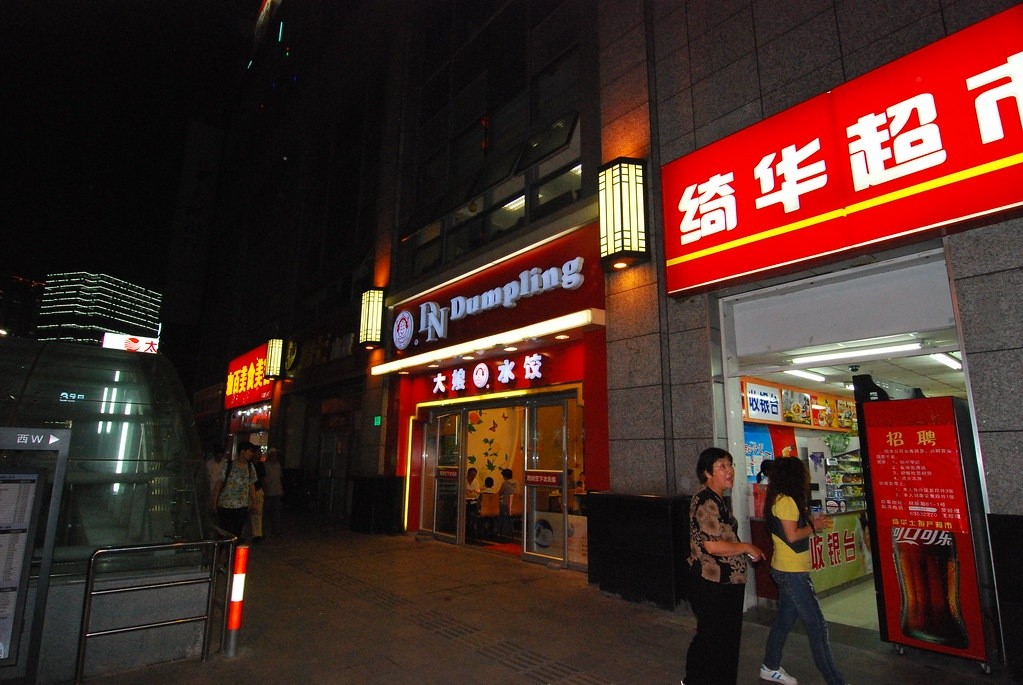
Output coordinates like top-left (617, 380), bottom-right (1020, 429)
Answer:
top-left (497, 493), bottom-right (529, 538)
top-left (467, 491), bottom-right (503, 541)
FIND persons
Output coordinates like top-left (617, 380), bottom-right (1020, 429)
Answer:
top-left (560, 469), bottom-right (586, 494)
top-left (261, 447), bottom-right (285, 537)
top-left (474, 477), bottom-right (496, 545)
top-left (238, 445), bottom-right (266, 542)
top-left (858, 516), bottom-right (873, 575)
top-left (464, 468), bottom-right (482, 504)
top-left (679, 447), bottom-right (767, 685)
top-left (756, 460), bottom-right (775, 485)
top-left (494, 469), bottom-right (519, 537)
top-left (209, 441), bottom-right (258, 571)
top-left (204, 447), bottom-right (229, 496)
top-left (760, 456), bottom-right (847, 685)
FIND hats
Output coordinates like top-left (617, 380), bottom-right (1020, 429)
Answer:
top-left (266, 448), bottom-right (281, 454)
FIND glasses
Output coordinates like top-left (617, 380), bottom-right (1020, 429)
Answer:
top-left (713, 463), bottom-right (736, 469)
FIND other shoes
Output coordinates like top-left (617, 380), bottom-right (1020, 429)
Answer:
top-left (253, 536), bottom-right (264, 542)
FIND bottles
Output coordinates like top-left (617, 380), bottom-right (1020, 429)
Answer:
top-left (826, 471), bottom-right (862, 500)
top-left (464, 489), bottom-right (478, 499)
top-left (552, 488), bottom-right (560, 495)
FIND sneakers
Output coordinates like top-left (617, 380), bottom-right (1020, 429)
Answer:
top-left (760, 664), bottom-right (798, 685)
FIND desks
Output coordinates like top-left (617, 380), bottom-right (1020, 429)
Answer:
top-left (466, 496), bottom-right (503, 530)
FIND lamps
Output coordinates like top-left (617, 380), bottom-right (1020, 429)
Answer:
top-left (597, 156), bottom-right (650, 273)
top-left (263, 337), bottom-right (287, 380)
top-left (358, 284), bottom-right (386, 351)
